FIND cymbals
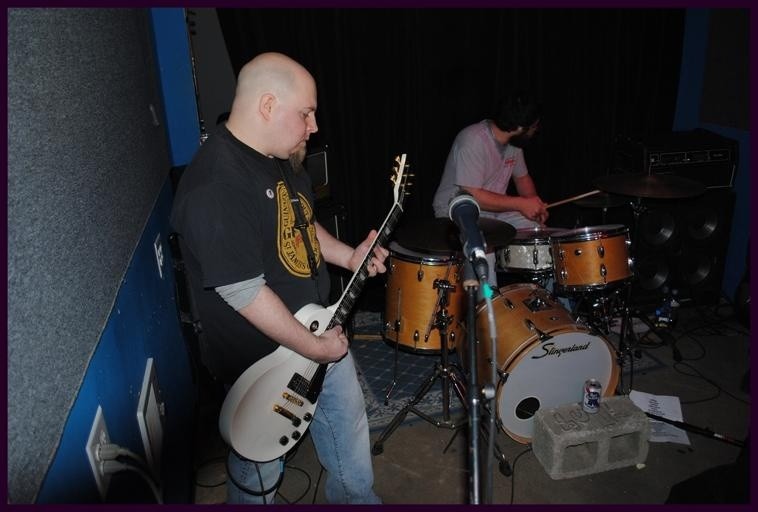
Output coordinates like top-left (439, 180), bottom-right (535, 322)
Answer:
top-left (391, 216), bottom-right (516, 254)
top-left (593, 172), bottom-right (693, 199)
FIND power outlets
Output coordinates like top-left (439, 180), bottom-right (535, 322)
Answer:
top-left (85, 404), bottom-right (116, 498)
top-left (154, 233), bottom-right (166, 280)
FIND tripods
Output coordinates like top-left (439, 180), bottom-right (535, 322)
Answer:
top-left (373, 279), bottom-right (514, 479)
top-left (573, 290), bottom-right (666, 365)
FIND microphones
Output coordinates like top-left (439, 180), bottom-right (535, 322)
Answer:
top-left (448, 190), bottom-right (496, 296)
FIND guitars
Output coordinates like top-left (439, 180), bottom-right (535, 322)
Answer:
top-left (219, 154), bottom-right (414, 462)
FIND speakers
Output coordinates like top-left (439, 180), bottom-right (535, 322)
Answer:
top-left (634, 193), bottom-right (737, 310)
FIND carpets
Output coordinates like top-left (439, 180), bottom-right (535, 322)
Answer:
top-left (348, 314), bottom-right (668, 433)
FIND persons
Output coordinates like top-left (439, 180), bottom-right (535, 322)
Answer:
top-left (430, 90), bottom-right (573, 316)
top-left (167, 52), bottom-right (391, 504)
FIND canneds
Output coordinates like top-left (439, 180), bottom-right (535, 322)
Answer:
top-left (582, 379), bottom-right (602, 413)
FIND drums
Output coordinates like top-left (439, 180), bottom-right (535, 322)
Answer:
top-left (551, 225), bottom-right (634, 291)
top-left (496, 227), bottom-right (572, 280)
top-left (478, 253), bottom-right (499, 304)
top-left (381, 242), bottom-right (476, 357)
top-left (453, 283), bottom-right (622, 444)
